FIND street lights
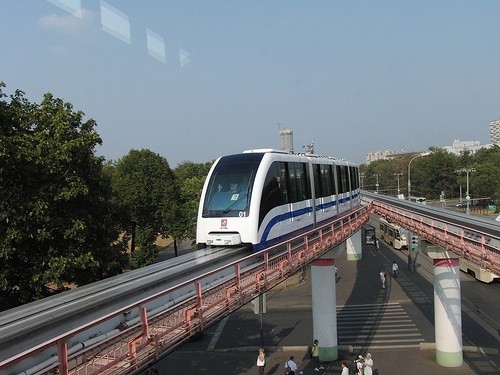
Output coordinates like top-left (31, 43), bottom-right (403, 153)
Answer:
top-left (408, 154), bottom-right (427, 201)
top-left (360, 175), bottom-right (365, 190)
top-left (373, 174), bottom-right (380, 194)
top-left (454, 168), bottom-right (477, 215)
top-left (394, 172), bottom-right (403, 195)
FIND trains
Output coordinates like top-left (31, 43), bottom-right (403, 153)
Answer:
top-left (195, 148), bottom-right (361, 257)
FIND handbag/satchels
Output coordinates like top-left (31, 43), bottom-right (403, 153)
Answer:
top-left (287, 367), bottom-right (291, 373)
top-left (310, 352), bottom-right (312, 359)
top-left (257, 354), bottom-right (265, 366)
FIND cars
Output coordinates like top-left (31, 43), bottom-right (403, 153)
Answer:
top-left (456, 203), bottom-right (461, 207)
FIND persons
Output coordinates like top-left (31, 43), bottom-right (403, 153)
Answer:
top-left (393, 261), bottom-right (398, 278)
top-left (380, 270), bottom-right (385, 288)
top-left (375, 238), bottom-right (379, 249)
top-left (311, 339), bottom-right (324, 371)
top-left (354, 353), bottom-right (373, 375)
top-left (258, 348), bottom-right (266, 375)
top-left (236, 175), bottom-right (249, 193)
top-left (150, 368), bottom-right (159, 375)
top-left (335, 265), bottom-right (338, 281)
top-left (342, 362), bottom-right (349, 375)
top-left (285, 356), bottom-right (303, 375)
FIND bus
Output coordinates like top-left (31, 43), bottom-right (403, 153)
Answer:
top-left (407, 196), bottom-right (427, 205)
top-left (379, 217), bottom-right (419, 251)
top-left (462, 200), bottom-right (474, 208)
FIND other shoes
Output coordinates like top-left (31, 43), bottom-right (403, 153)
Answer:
top-left (314, 367), bottom-right (319, 371)
top-left (319, 366), bottom-right (324, 370)
